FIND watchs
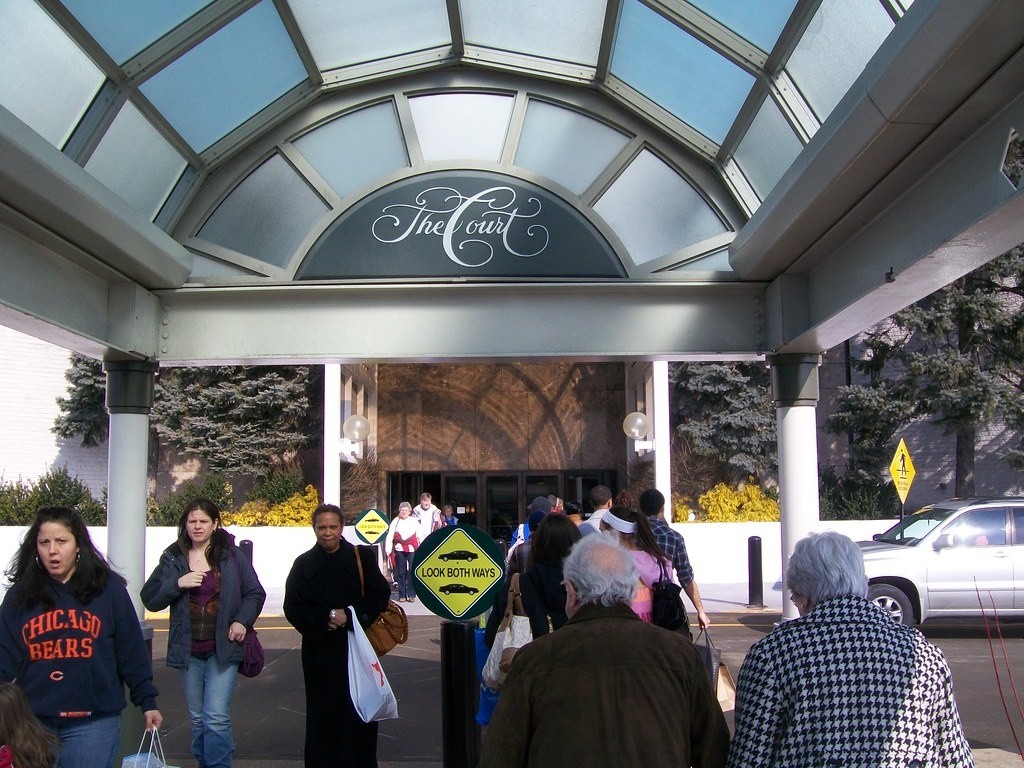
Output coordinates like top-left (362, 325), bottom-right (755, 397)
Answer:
top-left (330, 609), bottom-right (336, 618)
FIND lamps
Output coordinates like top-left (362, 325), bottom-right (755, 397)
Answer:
top-left (623, 412), bottom-right (653, 451)
top-left (340, 414), bottom-right (370, 457)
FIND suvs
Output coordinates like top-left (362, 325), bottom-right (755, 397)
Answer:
top-left (853, 495), bottom-right (1024, 633)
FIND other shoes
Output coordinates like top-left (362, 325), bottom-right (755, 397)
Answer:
top-left (399, 597), bottom-right (406, 602)
top-left (409, 597), bottom-right (414, 602)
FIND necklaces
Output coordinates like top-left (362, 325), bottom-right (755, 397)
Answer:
top-left (190, 550), bottom-right (212, 567)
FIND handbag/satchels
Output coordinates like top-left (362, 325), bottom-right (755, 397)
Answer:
top-left (364, 599), bottom-right (408, 657)
top-left (504, 524), bottom-right (525, 575)
top-left (692, 627), bottom-right (737, 712)
top-left (238, 627), bottom-right (265, 678)
top-left (346, 605), bottom-right (399, 723)
top-left (482, 573), bottom-right (533, 689)
top-left (651, 558), bottom-right (687, 631)
top-left (122, 724), bottom-right (180, 768)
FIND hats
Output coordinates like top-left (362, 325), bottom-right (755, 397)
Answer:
top-left (528, 510), bottom-right (545, 530)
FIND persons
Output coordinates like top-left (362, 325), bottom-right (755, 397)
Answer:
top-left (732, 532), bottom-right (978, 768)
top-left (481, 532), bottom-right (732, 768)
top-left (470, 482), bottom-right (710, 739)
top-left (0, 505), bottom-right (166, 768)
top-left (411, 493), bottom-right (442, 550)
top-left (385, 489), bottom-right (422, 603)
top-left (139, 497), bottom-right (268, 768)
top-left (437, 505), bottom-right (458, 530)
top-left (280, 503), bottom-right (409, 768)
